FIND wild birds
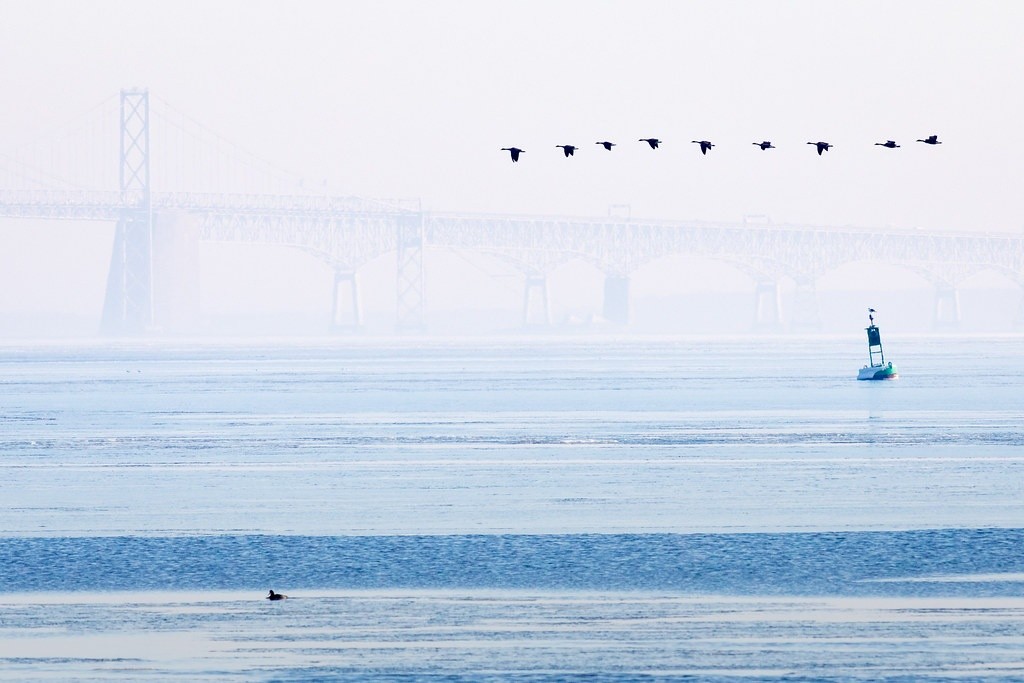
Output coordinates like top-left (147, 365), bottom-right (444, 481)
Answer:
top-left (875, 140), bottom-right (901, 149)
top-left (555, 145), bottom-right (579, 157)
top-left (268, 589), bottom-right (288, 602)
top-left (752, 140), bottom-right (775, 151)
top-left (501, 147), bottom-right (525, 163)
top-left (638, 138), bottom-right (662, 150)
top-left (806, 141), bottom-right (833, 156)
top-left (915, 134), bottom-right (942, 146)
top-left (690, 140), bottom-right (715, 156)
top-left (595, 141), bottom-right (616, 151)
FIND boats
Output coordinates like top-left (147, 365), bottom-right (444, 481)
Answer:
top-left (857, 307), bottom-right (898, 380)
